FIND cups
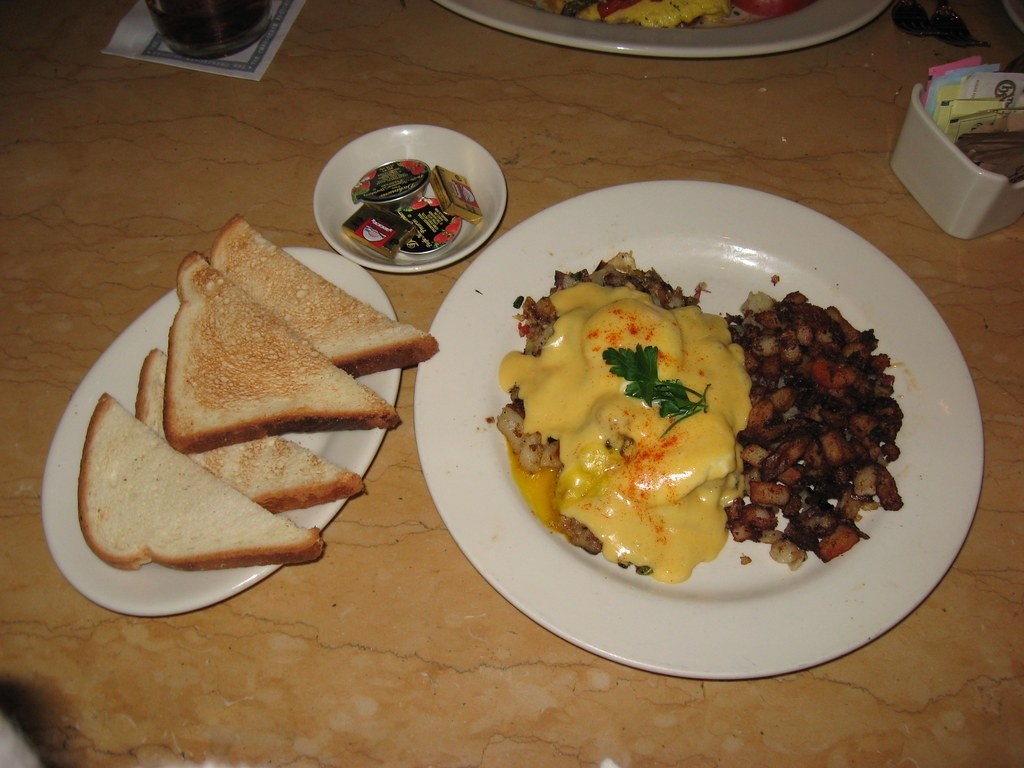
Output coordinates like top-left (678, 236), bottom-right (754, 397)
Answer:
top-left (145, 0), bottom-right (273, 60)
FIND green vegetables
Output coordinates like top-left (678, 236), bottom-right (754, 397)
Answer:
top-left (602, 342), bottom-right (711, 437)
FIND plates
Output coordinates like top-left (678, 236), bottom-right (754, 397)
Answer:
top-left (442, 1), bottom-right (894, 58)
top-left (38, 246), bottom-right (401, 618)
top-left (312, 122), bottom-right (508, 271)
top-left (414, 177), bottom-right (984, 680)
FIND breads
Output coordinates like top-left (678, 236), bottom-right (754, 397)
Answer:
top-left (163, 251), bottom-right (401, 454)
top-left (77, 392), bottom-right (324, 570)
top-left (206, 213), bottom-right (440, 378)
top-left (134, 347), bottom-right (365, 514)
